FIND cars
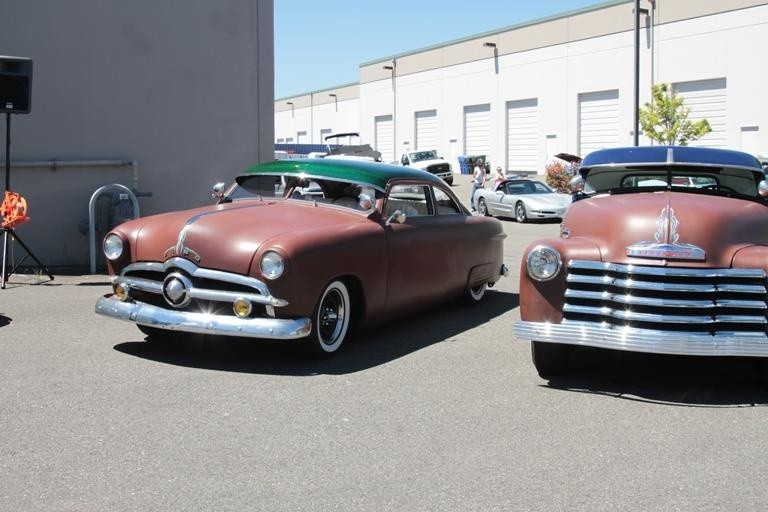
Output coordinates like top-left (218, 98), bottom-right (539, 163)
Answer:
top-left (99, 152), bottom-right (515, 362)
top-left (471, 179), bottom-right (572, 224)
top-left (308, 133), bottom-right (382, 162)
top-left (516, 145), bottom-right (768, 382)
top-left (551, 151), bottom-right (768, 200)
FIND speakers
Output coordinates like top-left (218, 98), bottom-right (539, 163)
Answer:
top-left (0, 55), bottom-right (32, 114)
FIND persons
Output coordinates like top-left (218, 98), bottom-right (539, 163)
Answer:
top-left (492, 167), bottom-right (505, 188)
top-left (469, 157), bottom-right (487, 213)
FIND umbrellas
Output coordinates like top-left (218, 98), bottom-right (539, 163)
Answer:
top-left (553, 152), bottom-right (581, 163)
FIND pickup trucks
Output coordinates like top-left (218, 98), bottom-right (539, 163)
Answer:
top-left (389, 152), bottom-right (454, 187)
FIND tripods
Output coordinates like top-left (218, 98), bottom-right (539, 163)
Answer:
top-left (0, 112), bottom-right (53, 290)
